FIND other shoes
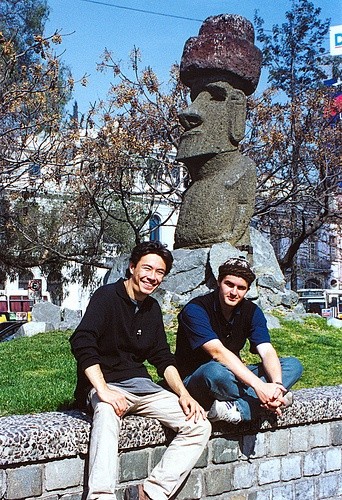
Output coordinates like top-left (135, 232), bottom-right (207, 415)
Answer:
top-left (129, 484), bottom-right (150, 500)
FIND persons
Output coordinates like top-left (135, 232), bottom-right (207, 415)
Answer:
top-left (155, 257), bottom-right (304, 425)
top-left (68, 240), bottom-right (212, 500)
top-left (329, 279), bottom-right (340, 308)
top-left (172, 13), bottom-right (264, 252)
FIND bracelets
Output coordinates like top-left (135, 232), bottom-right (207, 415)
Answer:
top-left (275, 382), bottom-right (284, 385)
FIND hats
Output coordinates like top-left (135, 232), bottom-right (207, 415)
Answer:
top-left (219, 258), bottom-right (256, 283)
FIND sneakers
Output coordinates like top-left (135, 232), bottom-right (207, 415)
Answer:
top-left (208, 400), bottom-right (241, 424)
